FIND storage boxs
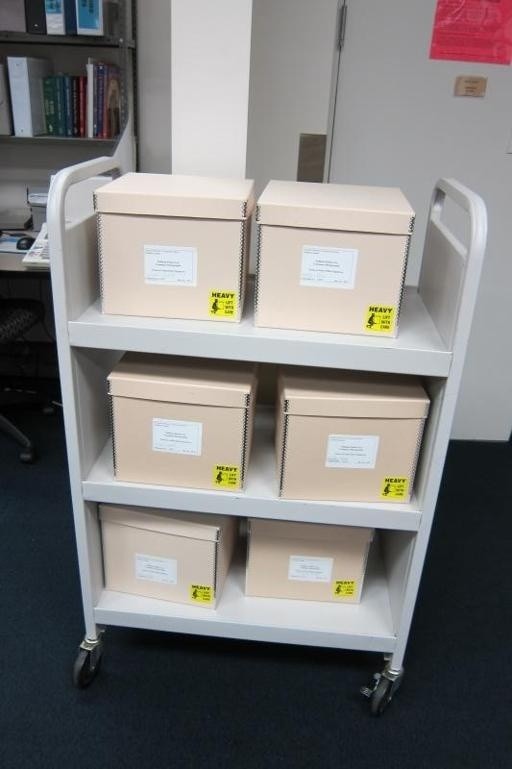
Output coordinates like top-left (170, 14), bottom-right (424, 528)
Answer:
top-left (97, 499), bottom-right (237, 607)
top-left (265, 363), bottom-right (438, 505)
top-left (96, 171), bottom-right (253, 324)
top-left (101, 352), bottom-right (256, 494)
top-left (242, 516), bottom-right (382, 606)
top-left (254, 181), bottom-right (423, 340)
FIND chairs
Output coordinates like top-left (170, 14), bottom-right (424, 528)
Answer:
top-left (1, 296), bottom-right (60, 462)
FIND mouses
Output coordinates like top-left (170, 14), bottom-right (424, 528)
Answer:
top-left (17, 237), bottom-right (35, 250)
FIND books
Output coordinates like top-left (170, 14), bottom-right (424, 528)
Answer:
top-left (22, 218), bottom-right (51, 268)
top-left (0, 0), bottom-right (127, 138)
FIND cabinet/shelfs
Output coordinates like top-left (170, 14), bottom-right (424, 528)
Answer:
top-left (1, 0), bottom-right (140, 278)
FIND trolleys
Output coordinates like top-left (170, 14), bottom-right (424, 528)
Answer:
top-left (35, 155), bottom-right (490, 719)
